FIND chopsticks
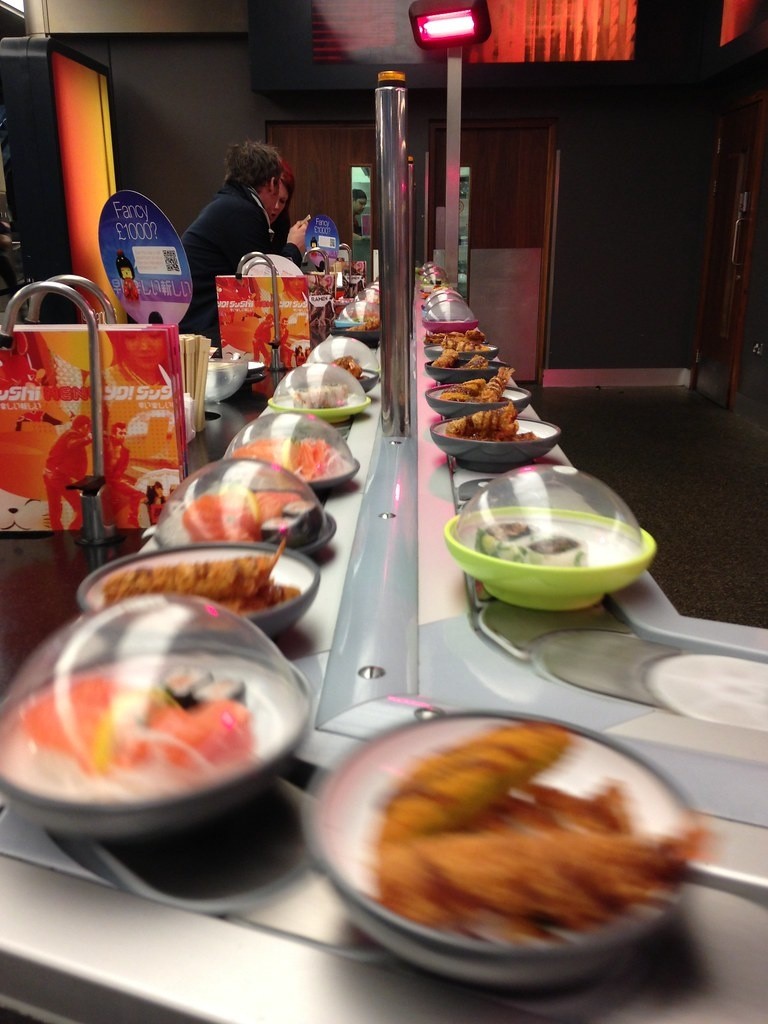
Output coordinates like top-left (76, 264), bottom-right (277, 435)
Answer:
top-left (300, 214), bottom-right (311, 225)
top-left (178, 334), bottom-right (212, 432)
top-left (330, 261), bottom-right (342, 276)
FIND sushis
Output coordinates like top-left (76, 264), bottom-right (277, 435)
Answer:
top-left (261, 501), bottom-right (321, 549)
top-left (290, 383), bottom-right (350, 410)
top-left (166, 666), bottom-right (246, 707)
top-left (475, 523), bottom-right (587, 567)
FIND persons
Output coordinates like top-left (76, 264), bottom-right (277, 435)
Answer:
top-left (264, 162), bottom-right (295, 243)
top-left (351, 188), bottom-right (368, 236)
top-left (43, 414), bottom-right (94, 531)
top-left (252, 277), bottom-right (309, 364)
top-left (80, 331), bottom-right (178, 460)
top-left (181, 139), bottom-right (308, 360)
top-left (103, 421), bottom-right (131, 525)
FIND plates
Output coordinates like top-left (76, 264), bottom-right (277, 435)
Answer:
top-left (0, 640), bottom-right (314, 841)
top-left (155, 509), bottom-right (337, 555)
top-left (424, 359), bottom-right (511, 384)
top-left (242, 362), bottom-right (267, 384)
top-left (355, 369), bottom-right (379, 394)
top-left (424, 344), bottom-right (499, 360)
top-left (329, 325), bottom-right (381, 348)
top-left (444, 506), bottom-right (657, 611)
top-left (305, 709), bottom-right (697, 993)
top-left (334, 318), bottom-right (365, 327)
top-left (77, 540), bottom-right (321, 640)
top-left (267, 396), bottom-right (371, 422)
top-left (422, 319), bottom-right (480, 333)
top-left (430, 417), bottom-right (562, 474)
top-left (226, 444), bottom-right (360, 490)
top-left (415, 266), bottom-right (451, 315)
top-left (425, 383), bottom-right (533, 418)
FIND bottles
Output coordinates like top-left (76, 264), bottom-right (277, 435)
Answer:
top-left (311, 237), bottom-right (317, 248)
top-left (116, 250), bottom-right (135, 280)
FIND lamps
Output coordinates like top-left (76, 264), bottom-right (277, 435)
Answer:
top-left (409, 1), bottom-right (492, 50)
